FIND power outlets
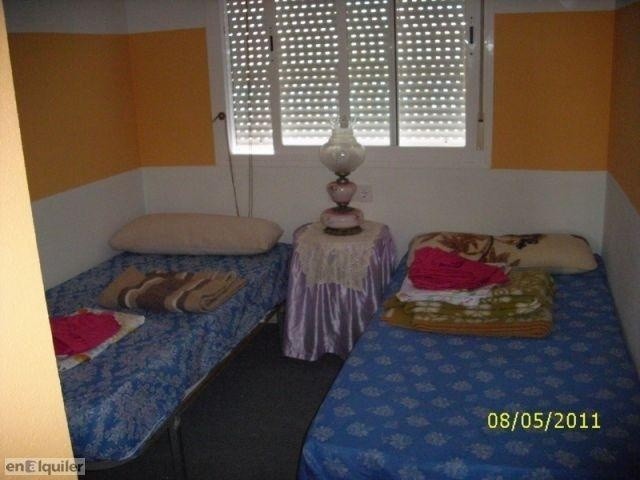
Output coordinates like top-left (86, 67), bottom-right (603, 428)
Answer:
top-left (360, 189), bottom-right (372, 202)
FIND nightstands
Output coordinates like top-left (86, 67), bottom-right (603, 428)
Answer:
top-left (281, 220), bottom-right (397, 361)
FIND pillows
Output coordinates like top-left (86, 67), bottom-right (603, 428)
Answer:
top-left (108, 214), bottom-right (284, 257)
top-left (406, 233), bottom-right (597, 275)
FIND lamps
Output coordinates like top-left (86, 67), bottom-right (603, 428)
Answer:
top-left (319, 127), bottom-right (366, 236)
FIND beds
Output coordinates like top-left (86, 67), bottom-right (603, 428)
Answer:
top-left (44, 241), bottom-right (292, 480)
top-left (297, 253), bottom-right (640, 480)
